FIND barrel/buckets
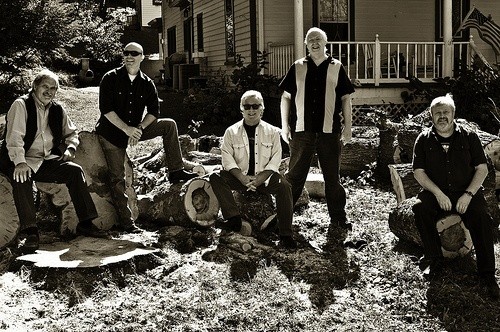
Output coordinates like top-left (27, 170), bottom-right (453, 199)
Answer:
top-left (179, 64), bottom-right (200, 88)
top-left (173, 64), bottom-right (179, 88)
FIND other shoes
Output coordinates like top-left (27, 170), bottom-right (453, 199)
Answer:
top-left (422, 266), bottom-right (441, 278)
top-left (280, 235), bottom-right (296, 248)
top-left (332, 220), bottom-right (352, 228)
top-left (216, 215), bottom-right (242, 232)
top-left (482, 278), bottom-right (499, 299)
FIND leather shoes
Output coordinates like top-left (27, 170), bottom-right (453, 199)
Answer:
top-left (25, 234), bottom-right (39, 249)
top-left (76, 222), bottom-right (107, 237)
top-left (123, 224), bottom-right (141, 233)
top-left (169, 170), bottom-right (199, 183)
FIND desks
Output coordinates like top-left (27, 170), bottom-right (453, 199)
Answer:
top-left (411, 54), bottom-right (440, 78)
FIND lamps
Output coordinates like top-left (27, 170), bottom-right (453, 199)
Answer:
top-left (184, 6), bottom-right (190, 17)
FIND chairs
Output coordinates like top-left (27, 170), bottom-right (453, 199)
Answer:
top-left (362, 40), bottom-right (397, 77)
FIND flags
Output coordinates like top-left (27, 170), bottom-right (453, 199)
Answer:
top-left (459, 6), bottom-right (500, 56)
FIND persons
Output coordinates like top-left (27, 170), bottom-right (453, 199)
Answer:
top-left (0, 69), bottom-right (108, 255)
top-left (208, 90), bottom-right (298, 249)
top-left (410, 95), bottom-right (500, 300)
top-left (279, 26), bottom-right (356, 229)
top-left (95, 41), bottom-right (199, 234)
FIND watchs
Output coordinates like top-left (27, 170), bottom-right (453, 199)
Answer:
top-left (136, 124), bottom-right (144, 133)
top-left (464, 191), bottom-right (473, 197)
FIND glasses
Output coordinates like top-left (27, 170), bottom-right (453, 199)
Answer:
top-left (123, 51), bottom-right (142, 56)
top-left (243, 104), bottom-right (261, 110)
